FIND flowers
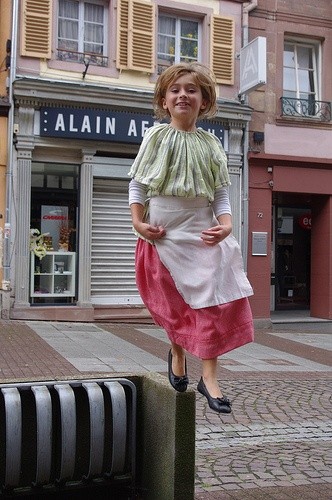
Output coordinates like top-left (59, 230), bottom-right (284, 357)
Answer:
top-left (169, 31), bottom-right (199, 63)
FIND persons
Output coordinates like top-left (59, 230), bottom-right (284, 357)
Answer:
top-left (128, 61), bottom-right (255, 413)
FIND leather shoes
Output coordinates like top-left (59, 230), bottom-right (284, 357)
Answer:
top-left (168, 347), bottom-right (190, 392)
top-left (197, 376), bottom-right (232, 413)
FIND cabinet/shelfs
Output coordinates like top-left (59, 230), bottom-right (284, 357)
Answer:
top-left (30, 251), bottom-right (75, 304)
